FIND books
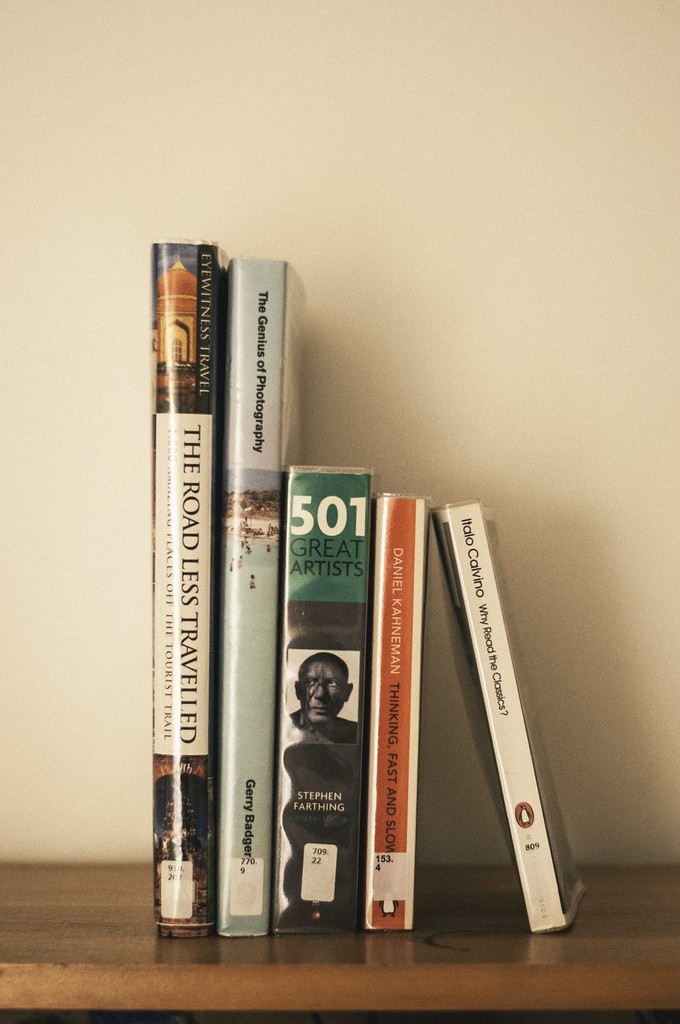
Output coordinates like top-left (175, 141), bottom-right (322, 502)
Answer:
top-left (218, 252), bottom-right (309, 938)
top-left (434, 495), bottom-right (586, 934)
top-left (362, 488), bottom-right (432, 931)
top-left (142, 238), bottom-right (224, 943)
top-left (273, 460), bottom-right (376, 935)
